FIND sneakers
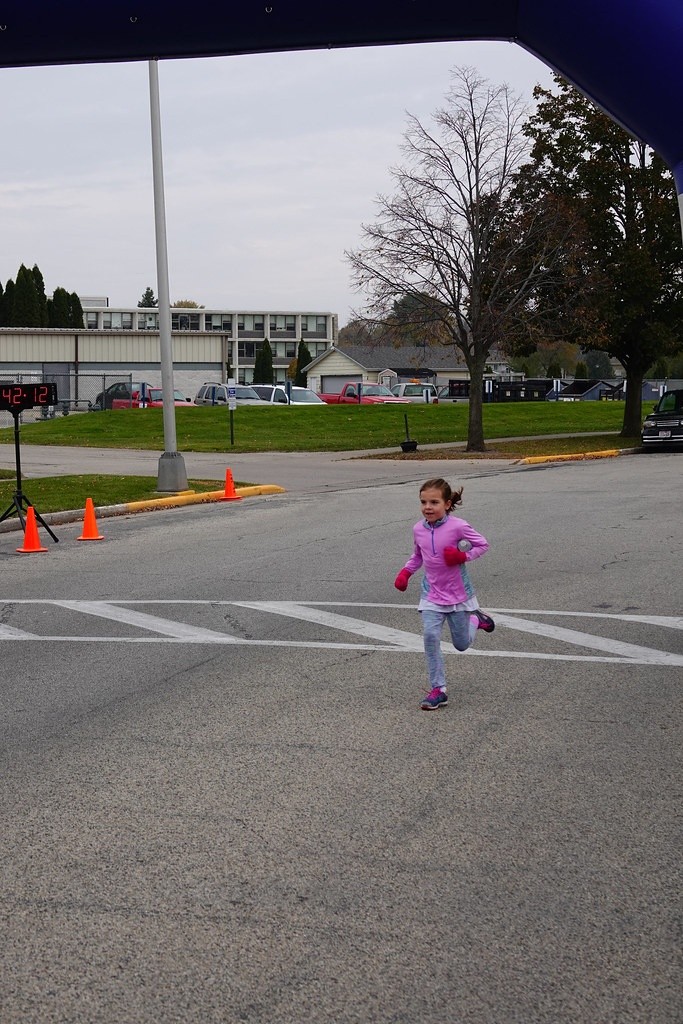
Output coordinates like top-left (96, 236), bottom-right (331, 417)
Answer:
top-left (421, 686), bottom-right (448, 709)
top-left (472, 608), bottom-right (496, 633)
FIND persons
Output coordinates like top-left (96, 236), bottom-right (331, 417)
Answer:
top-left (395, 478), bottom-right (496, 710)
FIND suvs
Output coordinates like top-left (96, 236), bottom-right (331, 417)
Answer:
top-left (194, 381), bottom-right (328, 406)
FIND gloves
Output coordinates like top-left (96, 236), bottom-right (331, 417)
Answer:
top-left (395, 568), bottom-right (412, 592)
top-left (444, 547), bottom-right (466, 566)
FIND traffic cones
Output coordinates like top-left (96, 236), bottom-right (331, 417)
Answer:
top-left (16, 506), bottom-right (48, 552)
top-left (219, 468), bottom-right (243, 500)
top-left (77, 498), bottom-right (104, 540)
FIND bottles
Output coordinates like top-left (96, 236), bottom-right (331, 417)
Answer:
top-left (458, 539), bottom-right (475, 564)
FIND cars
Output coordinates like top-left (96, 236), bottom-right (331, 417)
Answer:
top-left (390, 383), bottom-right (471, 403)
top-left (95, 381), bottom-right (162, 411)
top-left (641, 389), bottom-right (683, 445)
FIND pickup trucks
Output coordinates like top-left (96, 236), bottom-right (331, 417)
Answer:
top-left (313, 381), bottom-right (414, 404)
top-left (112, 388), bottom-right (197, 409)
